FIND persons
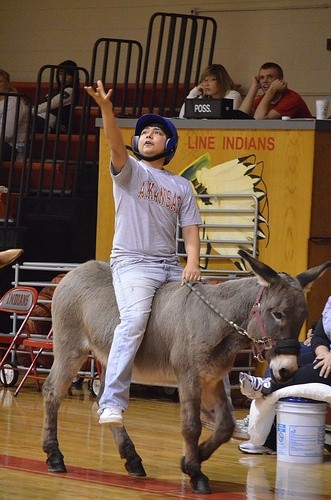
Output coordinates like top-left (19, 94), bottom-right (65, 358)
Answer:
top-left (30, 60), bottom-right (80, 134)
top-left (233, 296), bottom-right (331, 454)
top-left (84, 80), bottom-right (204, 428)
top-left (238, 61), bottom-right (313, 120)
top-left (179, 64), bottom-right (243, 120)
top-left (0, 69), bottom-right (28, 162)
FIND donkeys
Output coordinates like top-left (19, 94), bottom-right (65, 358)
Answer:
top-left (41, 247), bottom-right (330, 493)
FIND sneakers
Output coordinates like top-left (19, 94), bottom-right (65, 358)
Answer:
top-left (239, 372), bottom-right (272, 399)
top-left (99, 407), bottom-right (124, 428)
top-left (233, 416), bottom-right (249, 434)
top-left (238, 441), bottom-right (274, 454)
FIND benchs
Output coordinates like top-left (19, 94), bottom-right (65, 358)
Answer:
top-left (0, 82), bottom-right (192, 218)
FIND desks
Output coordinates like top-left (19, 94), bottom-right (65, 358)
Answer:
top-left (95, 119), bottom-right (331, 343)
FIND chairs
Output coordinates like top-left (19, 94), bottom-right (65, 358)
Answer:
top-left (0, 286), bottom-right (74, 396)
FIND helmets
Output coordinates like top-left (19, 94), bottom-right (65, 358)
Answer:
top-left (132, 113), bottom-right (178, 165)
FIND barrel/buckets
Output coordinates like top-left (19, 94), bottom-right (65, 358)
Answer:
top-left (273, 397), bottom-right (328, 464)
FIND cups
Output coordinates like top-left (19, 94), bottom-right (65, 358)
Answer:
top-left (316, 100), bottom-right (329, 120)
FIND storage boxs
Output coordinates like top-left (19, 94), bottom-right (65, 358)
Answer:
top-left (185, 99), bottom-right (234, 119)
